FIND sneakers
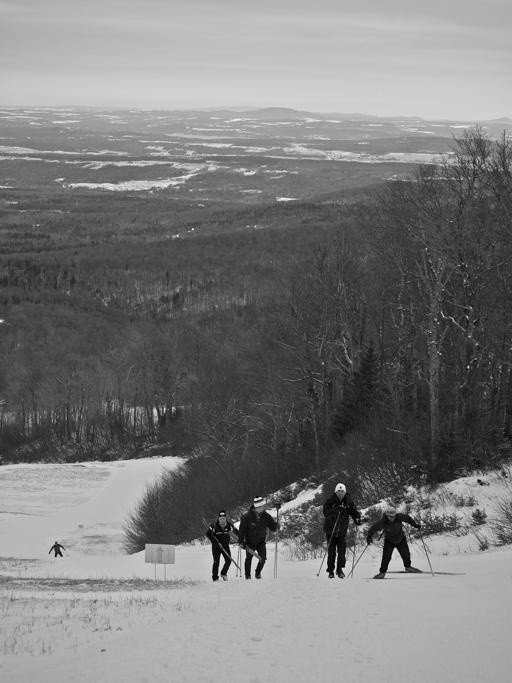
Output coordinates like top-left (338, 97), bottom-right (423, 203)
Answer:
top-left (329, 570), bottom-right (345, 578)
top-left (375, 573), bottom-right (384, 579)
top-left (214, 575), bottom-right (227, 583)
top-left (246, 573), bottom-right (260, 579)
top-left (405, 567), bottom-right (420, 572)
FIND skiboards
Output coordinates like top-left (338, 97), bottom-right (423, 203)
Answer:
top-left (229, 531), bottom-right (267, 563)
top-left (202, 518), bottom-right (242, 571)
top-left (362, 570), bottom-right (467, 580)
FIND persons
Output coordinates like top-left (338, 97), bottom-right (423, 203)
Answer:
top-left (366, 507), bottom-right (421, 579)
top-left (323, 483), bottom-right (361, 578)
top-left (206, 510), bottom-right (239, 582)
top-left (238, 496), bottom-right (280, 579)
top-left (48, 541), bottom-right (65, 557)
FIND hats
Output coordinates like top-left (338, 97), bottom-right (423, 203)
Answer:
top-left (386, 507), bottom-right (396, 516)
top-left (254, 496), bottom-right (266, 508)
top-left (335, 483), bottom-right (346, 493)
top-left (218, 511), bottom-right (226, 518)
